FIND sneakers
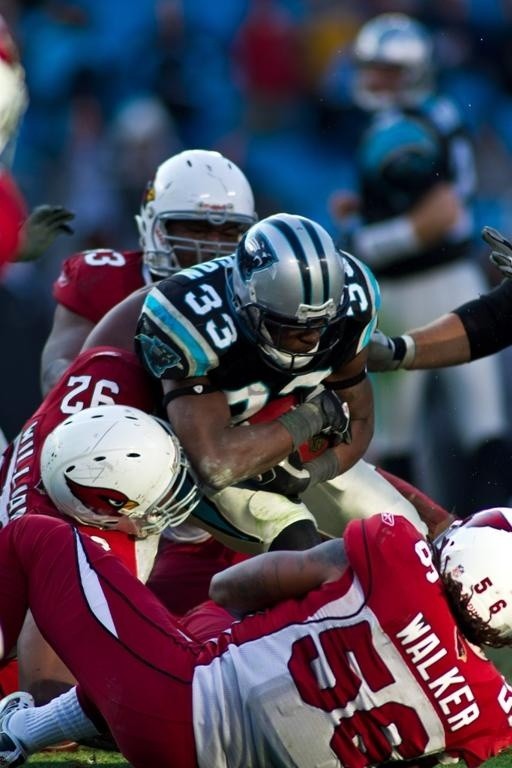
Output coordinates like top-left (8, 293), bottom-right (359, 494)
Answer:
top-left (0, 691), bottom-right (35, 768)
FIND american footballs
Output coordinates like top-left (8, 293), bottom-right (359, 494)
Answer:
top-left (239, 396), bottom-right (335, 463)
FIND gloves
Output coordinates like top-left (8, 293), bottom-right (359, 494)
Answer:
top-left (309, 387), bottom-right (352, 432)
top-left (365, 328), bottom-right (406, 373)
top-left (246, 450), bottom-right (310, 494)
top-left (483, 227), bottom-right (512, 279)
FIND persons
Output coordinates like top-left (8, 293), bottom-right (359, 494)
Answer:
top-left (362, 225), bottom-right (512, 371)
top-left (1, 15), bottom-right (76, 266)
top-left (40, 148), bottom-right (257, 399)
top-left (1, 214), bottom-right (512, 768)
top-left (328, 11), bottom-right (512, 511)
top-left (71, 2), bottom-right (363, 200)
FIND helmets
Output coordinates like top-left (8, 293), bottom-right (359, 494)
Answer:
top-left (431, 507), bottom-right (512, 649)
top-left (231, 212), bottom-right (344, 375)
top-left (136, 149), bottom-right (259, 277)
top-left (348, 10), bottom-right (432, 109)
top-left (40, 404), bottom-right (200, 539)
